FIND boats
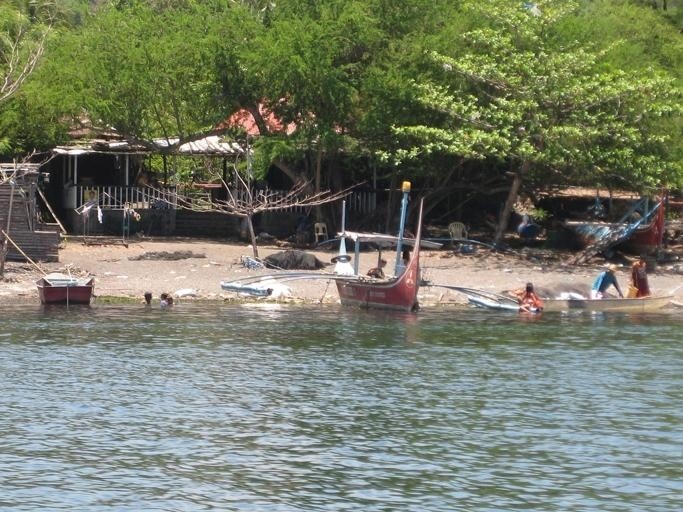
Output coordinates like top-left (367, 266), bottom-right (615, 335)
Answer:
top-left (562, 182), bottom-right (667, 259)
top-left (33, 272), bottom-right (98, 307)
top-left (495, 294), bottom-right (676, 316)
top-left (333, 197), bottom-right (428, 314)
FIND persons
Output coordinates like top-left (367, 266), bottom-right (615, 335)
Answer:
top-left (631, 253), bottom-right (652, 297)
top-left (591, 263), bottom-right (624, 299)
top-left (368, 258), bottom-right (388, 276)
top-left (399, 250), bottom-right (410, 269)
top-left (517, 282), bottom-right (544, 313)
top-left (86, 189), bottom-right (98, 208)
top-left (141, 293), bottom-right (173, 308)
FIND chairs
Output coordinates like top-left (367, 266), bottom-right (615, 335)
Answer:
top-left (448, 221), bottom-right (468, 248)
top-left (314, 223), bottom-right (328, 243)
